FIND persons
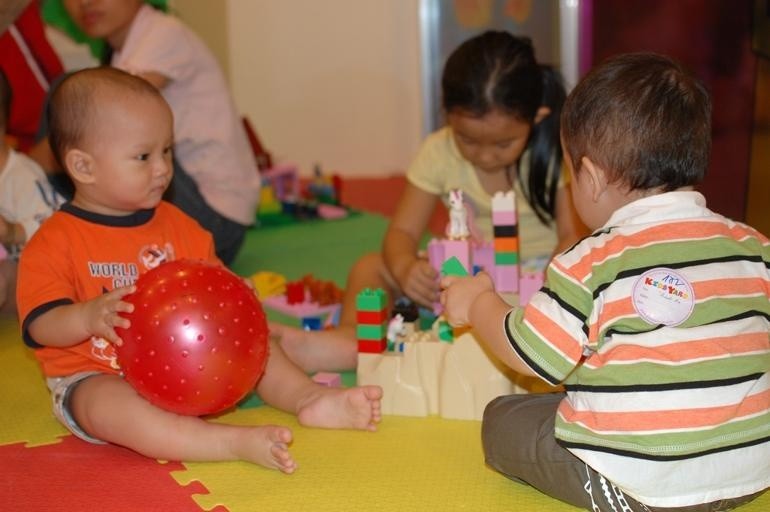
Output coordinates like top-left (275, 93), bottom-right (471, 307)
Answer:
top-left (0, 70), bottom-right (73, 320)
top-left (35, 0), bottom-right (261, 271)
top-left (17, 64), bottom-right (384, 475)
top-left (440, 50), bottom-right (770, 512)
top-left (258, 28), bottom-right (593, 376)
